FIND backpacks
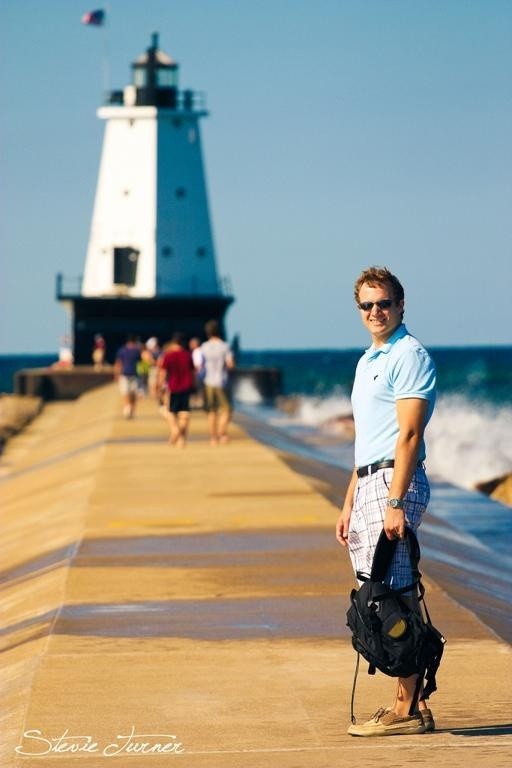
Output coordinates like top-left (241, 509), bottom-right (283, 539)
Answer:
top-left (345, 525), bottom-right (447, 701)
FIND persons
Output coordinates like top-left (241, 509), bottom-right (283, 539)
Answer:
top-left (92, 321), bottom-right (238, 446)
top-left (335, 268), bottom-right (436, 735)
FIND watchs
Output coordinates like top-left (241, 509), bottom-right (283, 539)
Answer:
top-left (387, 499), bottom-right (405, 508)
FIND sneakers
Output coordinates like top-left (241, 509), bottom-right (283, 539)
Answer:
top-left (420, 708), bottom-right (435, 732)
top-left (346, 707), bottom-right (425, 737)
top-left (123, 403), bottom-right (231, 450)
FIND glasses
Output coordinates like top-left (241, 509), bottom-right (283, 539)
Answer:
top-left (359, 299), bottom-right (396, 311)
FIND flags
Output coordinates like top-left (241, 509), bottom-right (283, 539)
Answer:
top-left (81, 8), bottom-right (105, 26)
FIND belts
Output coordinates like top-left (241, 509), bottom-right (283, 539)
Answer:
top-left (356, 458), bottom-right (422, 479)
